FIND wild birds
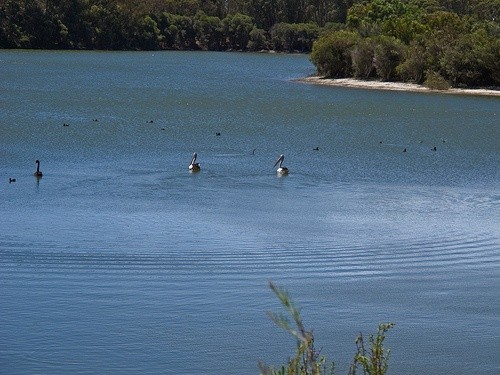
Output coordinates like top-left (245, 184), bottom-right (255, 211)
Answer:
top-left (34, 160), bottom-right (42, 175)
top-left (189, 153), bottom-right (200, 170)
top-left (8, 177), bottom-right (16, 182)
top-left (273, 154), bottom-right (288, 173)
